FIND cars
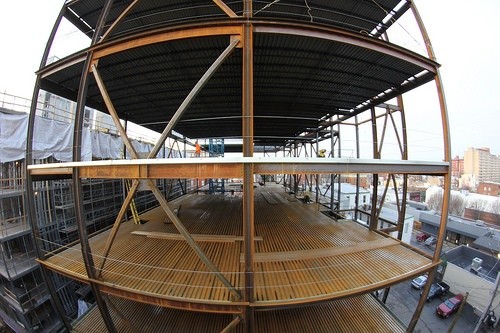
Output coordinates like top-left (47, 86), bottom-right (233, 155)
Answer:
top-left (416, 232), bottom-right (430, 240)
top-left (425, 236), bottom-right (438, 246)
top-left (410, 275), bottom-right (429, 290)
top-left (436, 293), bottom-right (464, 319)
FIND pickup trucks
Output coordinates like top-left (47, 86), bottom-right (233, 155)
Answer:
top-left (420, 282), bottom-right (450, 303)
